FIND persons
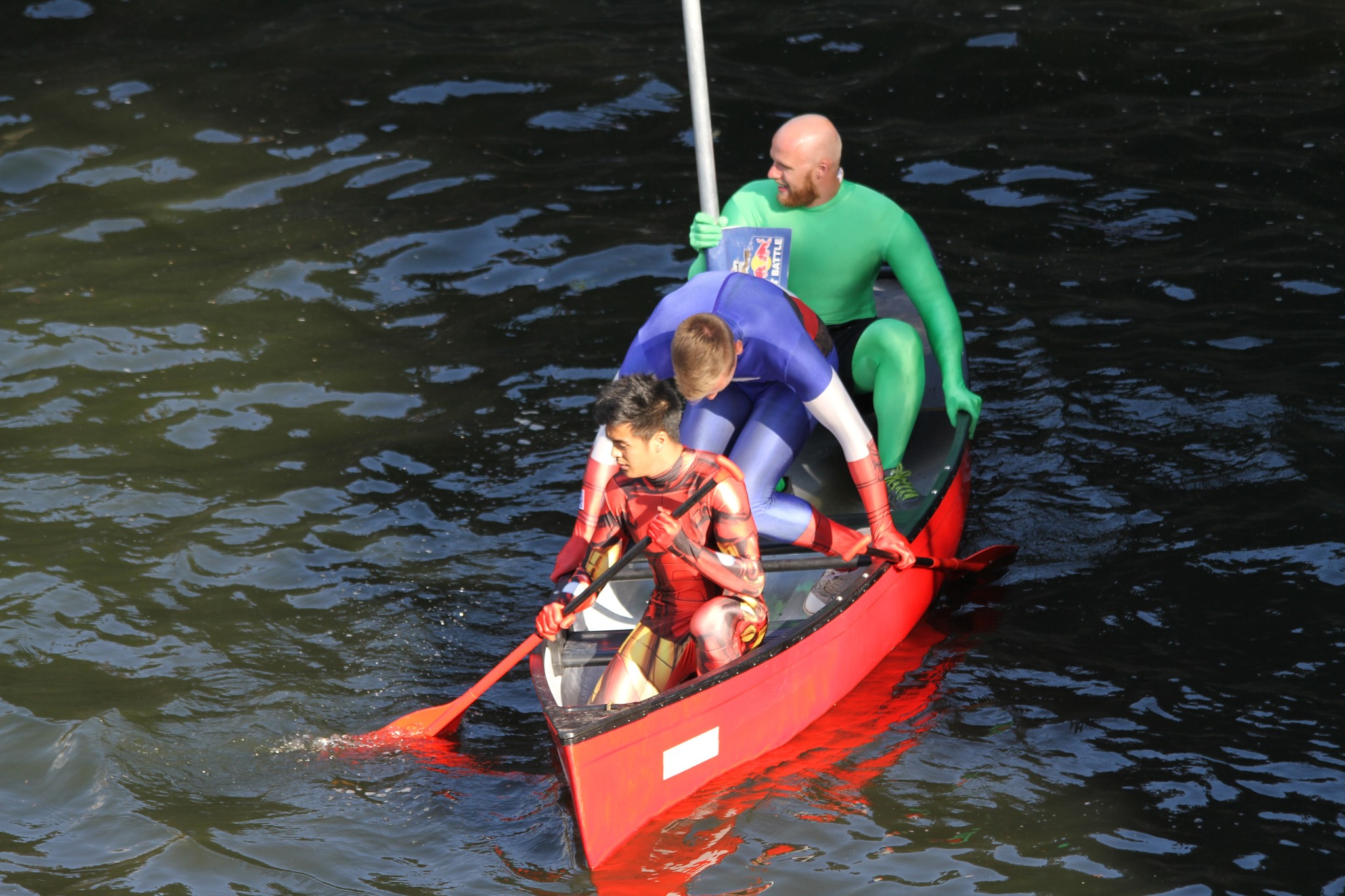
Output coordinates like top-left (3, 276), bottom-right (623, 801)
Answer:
top-left (687, 112), bottom-right (983, 514)
top-left (535, 370), bottom-right (769, 705)
top-left (550, 269), bottom-right (915, 615)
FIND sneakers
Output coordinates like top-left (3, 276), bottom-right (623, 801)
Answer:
top-left (803, 566), bottom-right (870, 615)
top-left (884, 462), bottom-right (925, 512)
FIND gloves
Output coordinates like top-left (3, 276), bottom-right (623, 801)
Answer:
top-left (549, 534), bottom-right (590, 585)
top-left (647, 506), bottom-right (687, 558)
top-left (689, 212), bottom-right (728, 259)
top-left (944, 378), bottom-right (984, 440)
top-left (869, 525), bottom-right (916, 572)
top-left (535, 601), bottom-right (577, 642)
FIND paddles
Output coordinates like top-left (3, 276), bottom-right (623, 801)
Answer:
top-left (843, 535), bottom-right (1019, 579)
top-left (381, 453), bottom-right (744, 743)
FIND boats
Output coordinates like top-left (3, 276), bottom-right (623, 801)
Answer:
top-left (527, 261), bottom-right (975, 869)
